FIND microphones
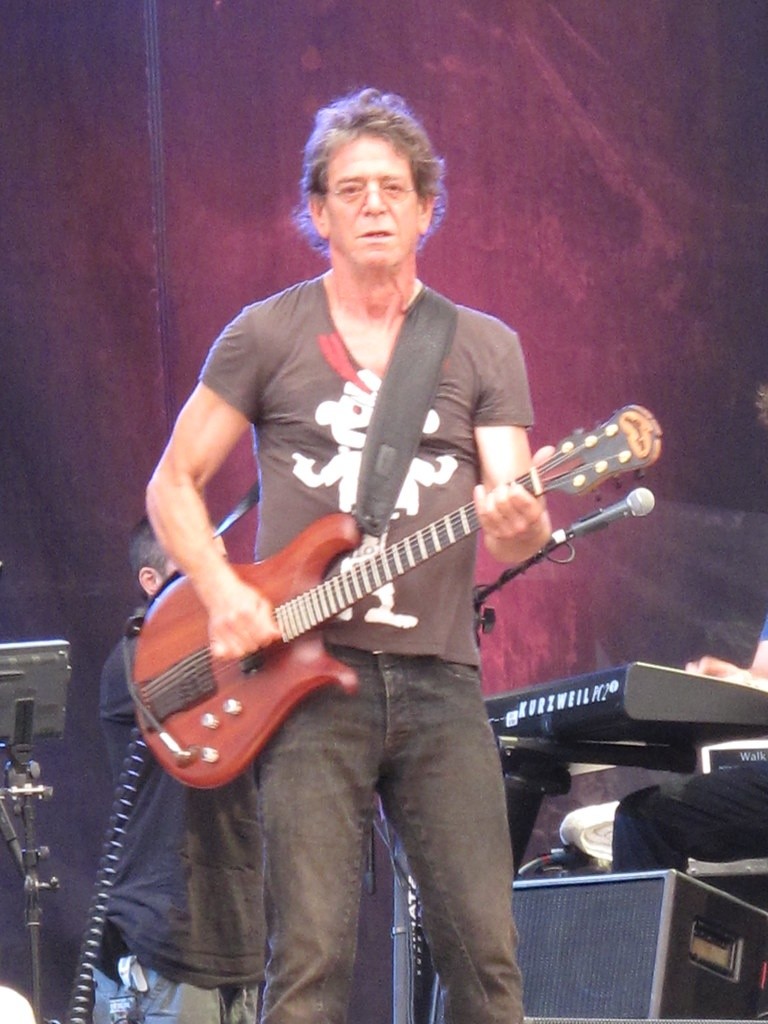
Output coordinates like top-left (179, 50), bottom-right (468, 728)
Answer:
top-left (551, 486), bottom-right (655, 545)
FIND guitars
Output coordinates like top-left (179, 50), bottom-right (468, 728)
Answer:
top-left (131, 404), bottom-right (666, 788)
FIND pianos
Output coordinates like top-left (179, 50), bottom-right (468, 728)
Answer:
top-left (483, 660), bottom-right (768, 879)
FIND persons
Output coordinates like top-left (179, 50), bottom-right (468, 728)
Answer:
top-left (612, 610), bottom-right (768, 878)
top-left (88, 519), bottom-right (273, 1023)
top-left (144, 87), bottom-right (551, 1024)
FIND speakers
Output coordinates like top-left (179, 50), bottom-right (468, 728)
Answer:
top-left (430, 867), bottom-right (767, 1022)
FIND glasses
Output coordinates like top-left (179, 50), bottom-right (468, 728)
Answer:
top-left (332, 184), bottom-right (417, 203)
